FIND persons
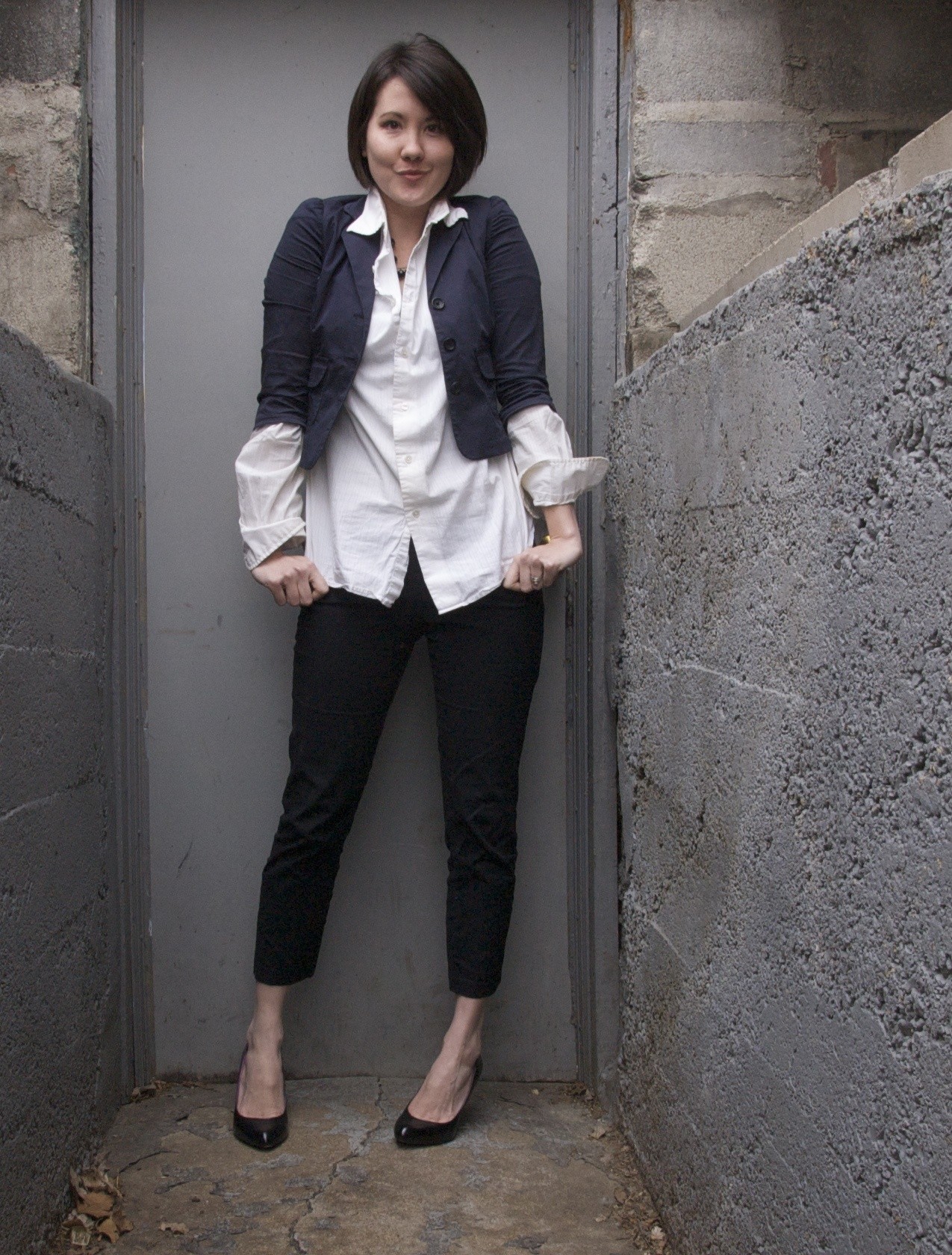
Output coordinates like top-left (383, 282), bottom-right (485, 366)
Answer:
top-left (232, 35), bottom-right (612, 1151)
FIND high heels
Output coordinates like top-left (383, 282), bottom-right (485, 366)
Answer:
top-left (396, 1051), bottom-right (484, 1147)
top-left (231, 1044), bottom-right (291, 1150)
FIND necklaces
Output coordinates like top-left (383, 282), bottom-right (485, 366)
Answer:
top-left (391, 237), bottom-right (406, 277)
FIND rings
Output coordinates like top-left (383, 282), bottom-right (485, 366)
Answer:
top-left (531, 575), bottom-right (543, 585)
